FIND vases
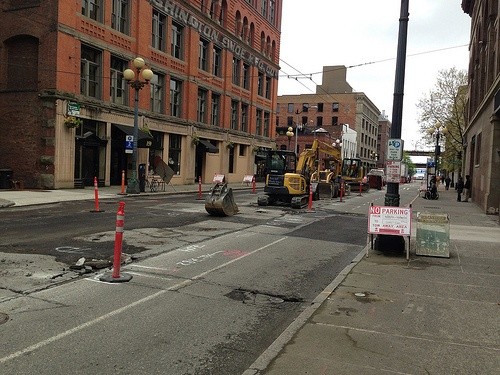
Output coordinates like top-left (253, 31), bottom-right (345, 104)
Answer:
top-left (66, 122), bottom-right (77, 128)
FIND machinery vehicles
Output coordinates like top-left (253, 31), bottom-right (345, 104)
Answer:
top-left (257, 137), bottom-right (370, 212)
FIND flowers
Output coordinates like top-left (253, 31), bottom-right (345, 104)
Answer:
top-left (64, 116), bottom-right (83, 128)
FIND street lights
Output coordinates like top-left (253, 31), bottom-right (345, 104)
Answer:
top-left (429, 122), bottom-right (447, 178)
top-left (286, 126), bottom-right (294, 170)
top-left (123, 57), bottom-right (153, 195)
top-left (295, 105), bottom-right (318, 163)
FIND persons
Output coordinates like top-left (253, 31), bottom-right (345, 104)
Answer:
top-left (441, 174), bottom-right (471, 203)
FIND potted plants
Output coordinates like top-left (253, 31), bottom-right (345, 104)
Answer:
top-left (191, 135), bottom-right (199, 145)
top-left (226, 141), bottom-right (234, 149)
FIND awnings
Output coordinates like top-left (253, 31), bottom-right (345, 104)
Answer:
top-left (113, 124), bottom-right (151, 142)
top-left (196, 138), bottom-right (220, 154)
